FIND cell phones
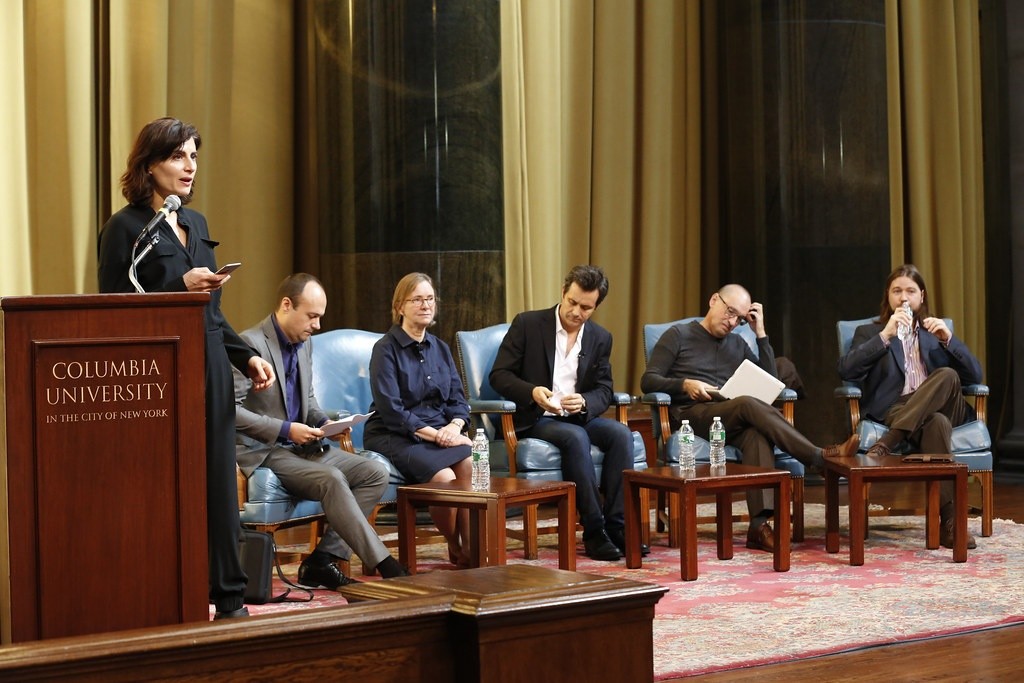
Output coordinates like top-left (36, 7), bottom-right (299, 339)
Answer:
top-left (214, 263), bottom-right (242, 274)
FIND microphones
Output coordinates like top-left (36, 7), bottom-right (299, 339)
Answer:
top-left (134, 194), bottom-right (182, 247)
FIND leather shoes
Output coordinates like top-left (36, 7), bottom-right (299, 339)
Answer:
top-left (296, 559), bottom-right (359, 590)
top-left (818, 433), bottom-right (862, 479)
top-left (746, 522), bottom-right (794, 554)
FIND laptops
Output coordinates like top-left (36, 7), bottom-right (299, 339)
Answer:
top-left (706, 359), bottom-right (786, 405)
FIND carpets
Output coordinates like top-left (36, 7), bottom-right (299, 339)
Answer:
top-left (209, 500), bottom-right (1024, 681)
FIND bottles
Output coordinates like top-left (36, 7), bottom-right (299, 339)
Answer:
top-left (896, 302), bottom-right (913, 341)
top-left (679, 420), bottom-right (696, 470)
top-left (709, 417), bottom-right (726, 467)
top-left (471, 428), bottom-right (490, 492)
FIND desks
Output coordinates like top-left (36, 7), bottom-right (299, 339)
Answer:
top-left (623, 458), bottom-right (793, 581)
top-left (395, 476), bottom-right (579, 577)
top-left (823, 454), bottom-right (970, 566)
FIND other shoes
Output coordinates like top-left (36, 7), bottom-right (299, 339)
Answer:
top-left (866, 442), bottom-right (889, 455)
top-left (939, 518), bottom-right (977, 548)
top-left (582, 523), bottom-right (621, 561)
top-left (609, 526), bottom-right (648, 556)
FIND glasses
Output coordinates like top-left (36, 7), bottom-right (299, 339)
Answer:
top-left (403, 298), bottom-right (436, 307)
top-left (718, 294), bottom-right (746, 322)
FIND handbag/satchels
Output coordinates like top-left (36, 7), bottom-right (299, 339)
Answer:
top-left (237, 528), bottom-right (313, 605)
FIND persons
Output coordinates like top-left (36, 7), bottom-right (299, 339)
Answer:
top-left (363, 272), bottom-right (475, 568)
top-left (96, 118), bottom-right (276, 621)
top-left (640, 283), bottom-right (862, 554)
top-left (229, 272), bottom-right (413, 590)
top-left (488, 265), bottom-right (651, 561)
top-left (835, 264), bottom-right (984, 549)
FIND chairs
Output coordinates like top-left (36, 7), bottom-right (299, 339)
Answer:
top-left (456, 323), bottom-right (648, 553)
top-left (312, 327), bottom-right (472, 576)
top-left (232, 426), bottom-right (358, 583)
top-left (834, 316), bottom-right (995, 541)
top-left (641, 317), bottom-right (810, 550)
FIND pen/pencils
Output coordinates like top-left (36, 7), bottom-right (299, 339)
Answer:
top-left (313, 425), bottom-right (323, 452)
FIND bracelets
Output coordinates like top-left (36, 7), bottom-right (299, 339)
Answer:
top-left (451, 419), bottom-right (462, 429)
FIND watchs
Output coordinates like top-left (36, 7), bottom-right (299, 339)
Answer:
top-left (580, 397), bottom-right (586, 411)
